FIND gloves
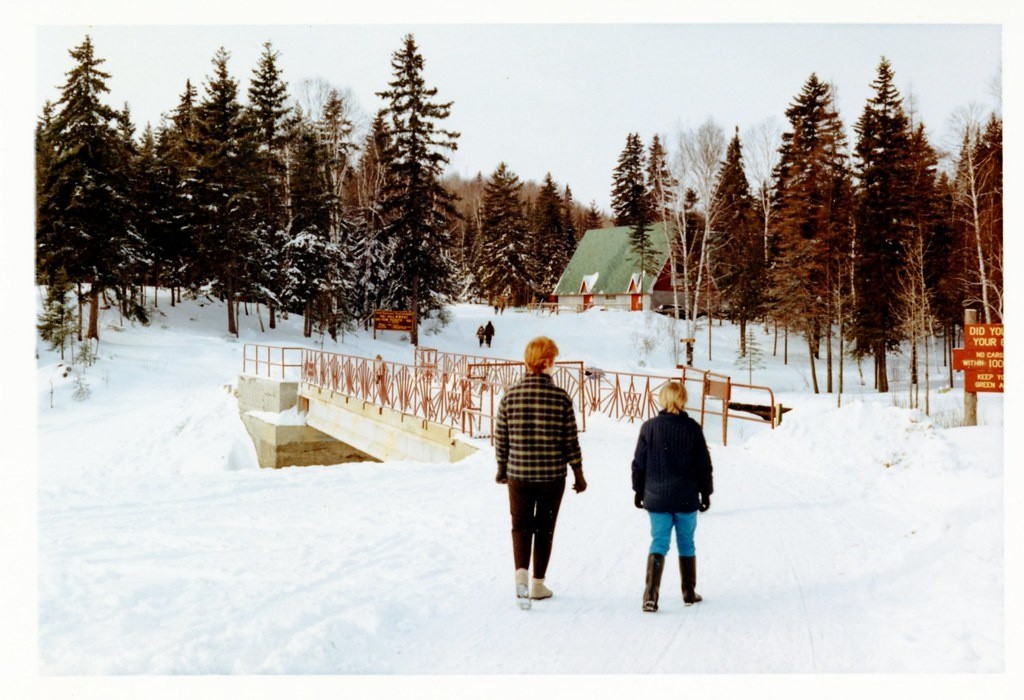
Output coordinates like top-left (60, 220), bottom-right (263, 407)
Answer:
top-left (572, 471), bottom-right (587, 494)
top-left (698, 492), bottom-right (711, 513)
top-left (494, 461), bottom-right (508, 485)
top-left (634, 492), bottom-right (645, 508)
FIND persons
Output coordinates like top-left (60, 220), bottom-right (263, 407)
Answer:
top-left (476, 321), bottom-right (494, 348)
top-left (494, 293), bottom-right (513, 316)
top-left (632, 383), bottom-right (714, 612)
top-left (496, 337), bottom-right (587, 610)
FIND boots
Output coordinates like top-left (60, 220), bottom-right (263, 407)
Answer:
top-left (527, 576), bottom-right (556, 600)
top-left (678, 556), bottom-right (703, 607)
top-left (642, 553), bottom-right (665, 612)
top-left (513, 568), bottom-right (531, 611)
